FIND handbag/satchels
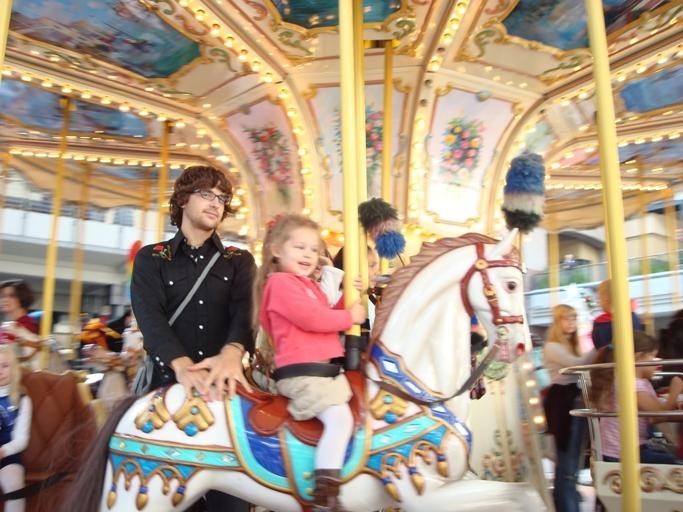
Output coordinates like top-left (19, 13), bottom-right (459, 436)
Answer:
top-left (133, 354), bottom-right (157, 396)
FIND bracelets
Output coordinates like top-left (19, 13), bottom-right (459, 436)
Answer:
top-left (224, 342), bottom-right (244, 358)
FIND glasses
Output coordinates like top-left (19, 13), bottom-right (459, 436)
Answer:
top-left (184, 188), bottom-right (229, 203)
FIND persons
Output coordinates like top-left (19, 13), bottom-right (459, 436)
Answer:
top-left (102, 310), bottom-right (134, 355)
top-left (0, 277), bottom-right (41, 345)
top-left (518, 275), bottom-right (682, 510)
top-left (249, 210), bottom-right (370, 510)
top-left (0, 344), bottom-right (34, 511)
top-left (332, 241), bottom-right (380, 337)
top-left (126, 163), bottom-right (264, 511)
